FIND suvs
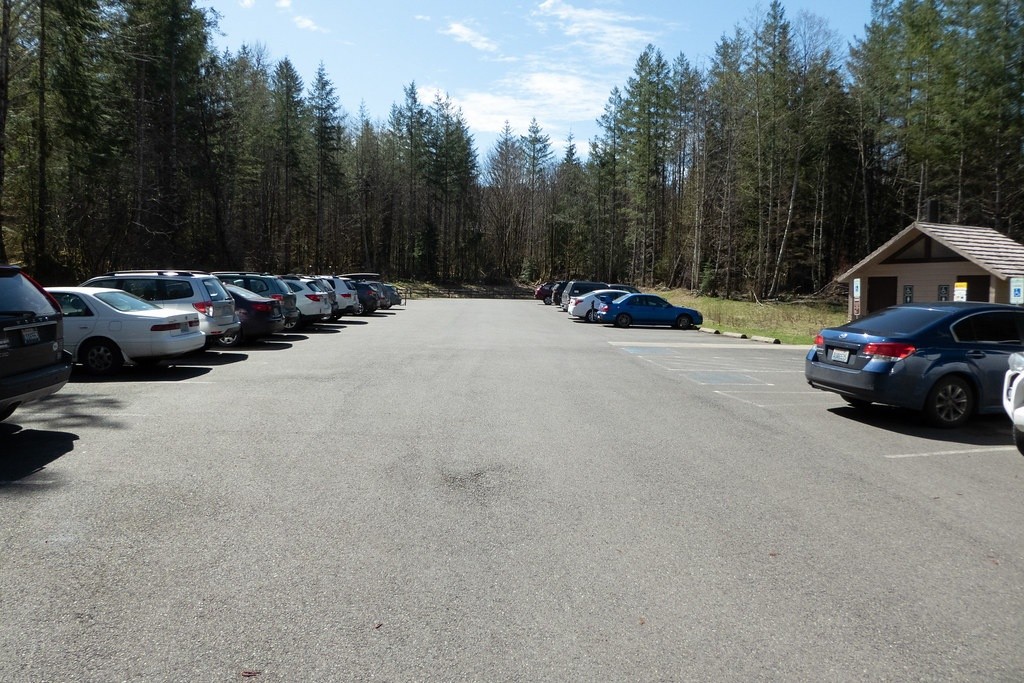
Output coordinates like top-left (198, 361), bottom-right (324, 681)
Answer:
top-left (0, 264), bottom-right (74, 424)
top-left (560, 279), bottom-right (642, 313)
top-left (207, 271), bottom-right (402, 347)
top-left (65, 269), bottom-right (242, 354)
top-left (534, 281), bottom-right (555, 305)
top-left (551, 280), bottom-right (568, 306)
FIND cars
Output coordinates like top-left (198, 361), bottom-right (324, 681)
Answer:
top-left (41, 287), bottom-right (206, 382)
top-left (567, 289), bottom-right (639, 323)
top-left (805, 301), bottom-right (1024, 429)
top-left (597, 293), bottom-right (703, 330)
